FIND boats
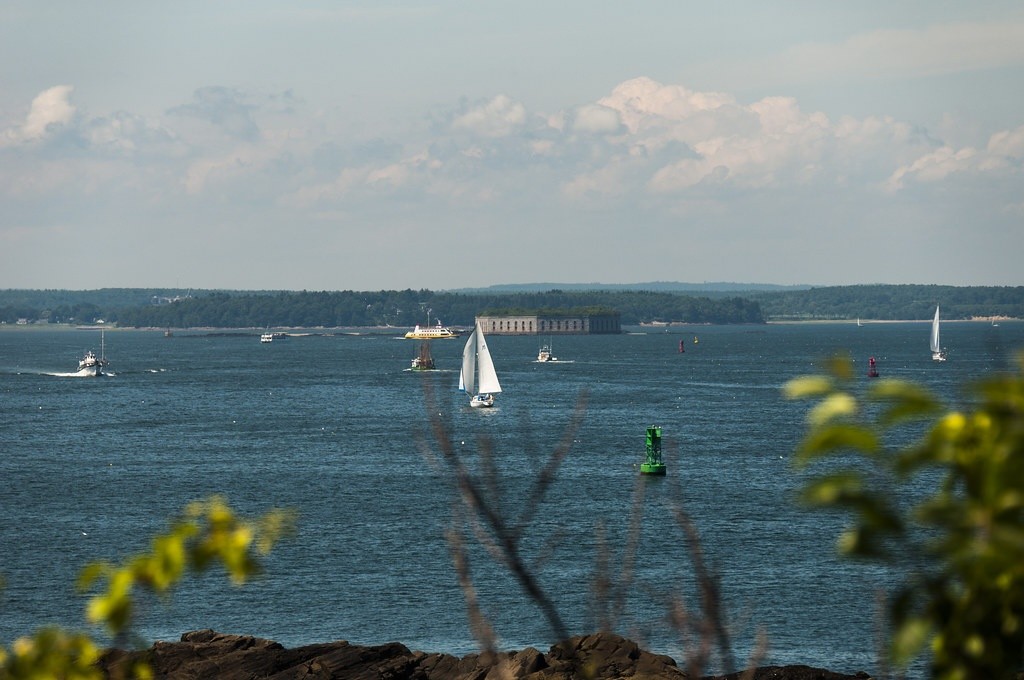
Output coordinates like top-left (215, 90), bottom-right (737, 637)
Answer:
top-left (76, 328), bottom-right (110, 376)
top-left (261, 331), bottom-right (291, 343)
top-left (641, 424), bottom-right (668, 474)
top-left (404, 308), bottom-right (461, 340)
top-left (866, 357), bottom-right (879, 377)
top-left (537, 332), bottom-right (558, 363)
top-left (402, 340), bottom-right (450, 372)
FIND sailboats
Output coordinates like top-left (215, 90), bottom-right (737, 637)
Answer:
top-left (458, 320), bottom-right (502, 407)
top-left (929, 302), bottom-right (946, 362)
